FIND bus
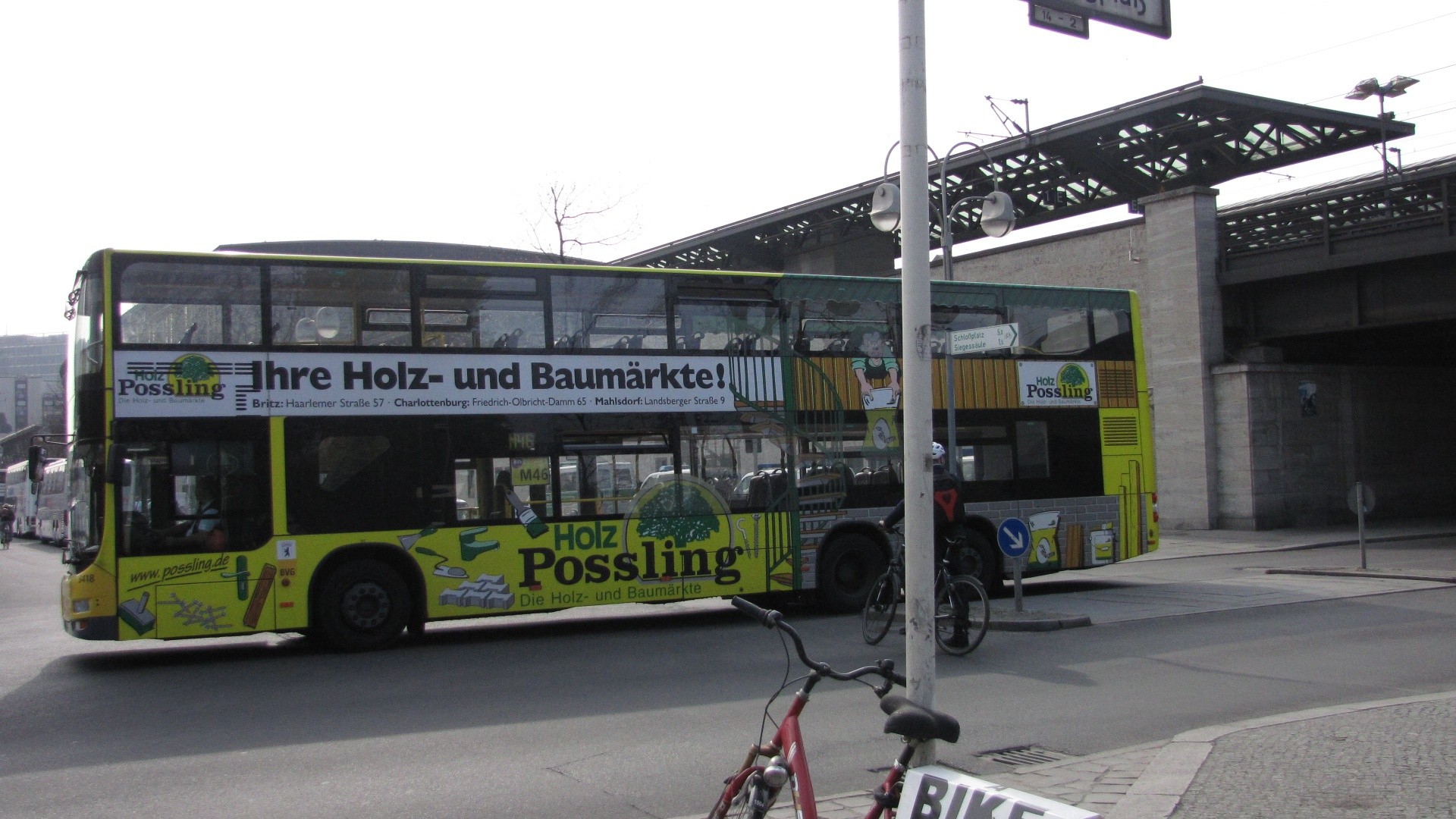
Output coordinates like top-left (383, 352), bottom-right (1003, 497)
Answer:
top-left (36, 458), bottom-right (68, 546)
top-left (28, 247), bottom-right (1161, 654)
top-left (0, 469), bottom-right (6, 506)
top-left (4, 460), bottom-right (39, 537)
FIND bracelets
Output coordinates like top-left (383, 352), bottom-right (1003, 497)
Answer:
top-left (176, 537), bottom-right (182, 544)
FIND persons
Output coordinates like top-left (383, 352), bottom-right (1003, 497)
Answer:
top-left (879, 441), bottom-right (972, 647)
top-left (0, 504), bottom-right (16, 544)
top-left (149, 476), bottom-right (220, 547)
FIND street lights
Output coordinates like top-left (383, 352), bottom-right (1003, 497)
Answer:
top-left (870, 138), bottom-right (1018, 481)
top-left (1344, 75), bottom-right (1420, 219)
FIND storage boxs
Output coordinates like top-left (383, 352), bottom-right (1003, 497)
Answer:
top-left (1210, 361), bottom-right (1350, 531)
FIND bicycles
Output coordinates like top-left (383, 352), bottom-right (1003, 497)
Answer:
top-left (860, 522), bottom-right (992, 656)
top-left (708, 596), bottom-right (961, 819)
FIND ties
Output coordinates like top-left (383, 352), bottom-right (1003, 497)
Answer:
top-left (192, 506), bottom-right (210, 534)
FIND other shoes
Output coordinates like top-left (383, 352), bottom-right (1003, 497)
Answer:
top-left (942, 635), bottom-right (969, 648)
top-left (899, 627), bottom-right (906, 635)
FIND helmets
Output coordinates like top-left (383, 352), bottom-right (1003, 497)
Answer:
top-left (932, 442), bottom-right (945, 466)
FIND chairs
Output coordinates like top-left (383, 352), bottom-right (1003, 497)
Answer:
top-left (176, 321), bottom-right (945, 356)
